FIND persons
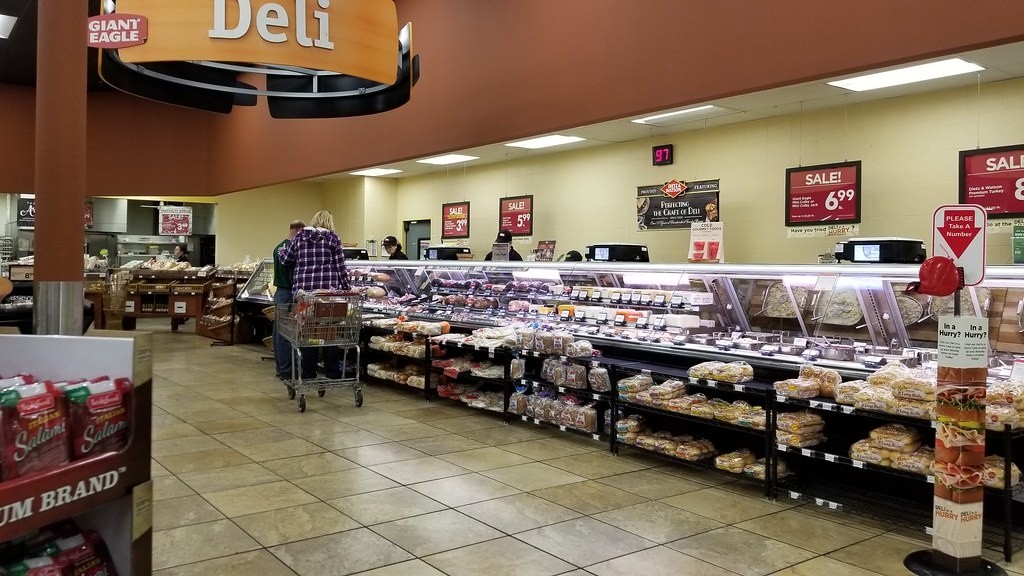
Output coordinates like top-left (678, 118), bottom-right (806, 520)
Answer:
top-left (277, 210), bottom-right (352, 379)
top-left (273, 219), bottom-right (305, 381)
top-left (565, 250), bottom-right (582, 262)
top-left (484, 230), bottom-right (524, 261)
top-left (382, 235), bottom-right (408, 261)
top-left (173, 243), bottom-right (191, 264)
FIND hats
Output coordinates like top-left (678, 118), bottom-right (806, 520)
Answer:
top-left (381, 236), bottom-right (397, 246)
top-left (564, 250), bottom-right (582, 261)
top-left (495, 229), bottom-right (512, 243)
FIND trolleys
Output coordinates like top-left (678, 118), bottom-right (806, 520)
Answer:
top-left (275, 286), bottom-right (365, 413)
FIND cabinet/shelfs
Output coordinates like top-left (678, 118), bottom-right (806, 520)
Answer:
top-left (0, 333), bottom-right (152, 576)
top-left (81, 265), bottom-right (254, 339)
top-left (358, 313), bottom-right (936, 537)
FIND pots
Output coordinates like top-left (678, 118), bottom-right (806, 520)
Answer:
top-left (779, 344), bottom-right (805, 355)
top-left (817, 344), bottom-right (857, 360)
top-left (690, 332), bottom-right (721, 347)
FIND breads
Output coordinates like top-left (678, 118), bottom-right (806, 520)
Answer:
top-left (350, 269), bottom-right (625, 437)
top-left (616, 360), bottom-right (1024, 491)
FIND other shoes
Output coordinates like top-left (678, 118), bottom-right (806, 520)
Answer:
top-left (280, 373), bottom-right (298, 380)
top-left (276, 370), bottom-right (281, 376)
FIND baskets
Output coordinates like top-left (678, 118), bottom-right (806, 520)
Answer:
top-left (210, 282), bottom-right (235, 297)
top-left (209, 302), bottom-right (233, 318)
top-left (263, 336), bottom-right (274, 351)
top-left (261, 306), bottom-right (276, 321)
top-left (208, 319), bottom-right (232, 337)
